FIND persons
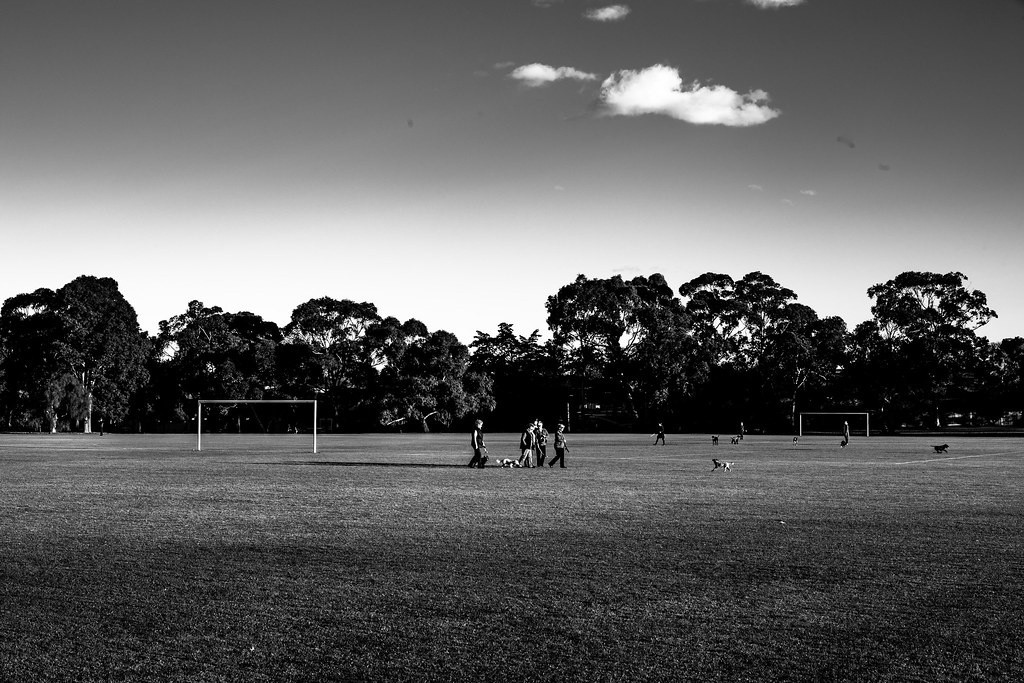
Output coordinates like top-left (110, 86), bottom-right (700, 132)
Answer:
top-left (653, 423), bottom-right (666, 446)
top-left (844, 421), bottom-right (849, 446)
top-left (548, 424), bottom-right (570, 468)
top-left (517, 417), bottom-right (549, 469)
top-left (738, 422), bottom-right (747, 440)
top-left (467, 420), bottom-right (488, 469)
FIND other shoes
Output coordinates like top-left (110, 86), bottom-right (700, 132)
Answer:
top-left (560, 466), bottom-right (567, 468)
top-left (528, 466), bottom-right (535, 468)
top-left (548, 463), bottom-right (553, 468)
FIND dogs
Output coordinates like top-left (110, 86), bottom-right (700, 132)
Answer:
top-left (730, 434), bottom-right (741, 444)
top-left (930, 444), bottom-right (949, 454)
top-left (792, 436), bottom-right (798, 446)
top-left (499, 458), bottom-right (520, 468)
top-left (840, 440), bottom-right (848, 448)
top-left (711, 434), bottom-right (720, 446)
top-left (711, 459), bottom-right (734, 472)
